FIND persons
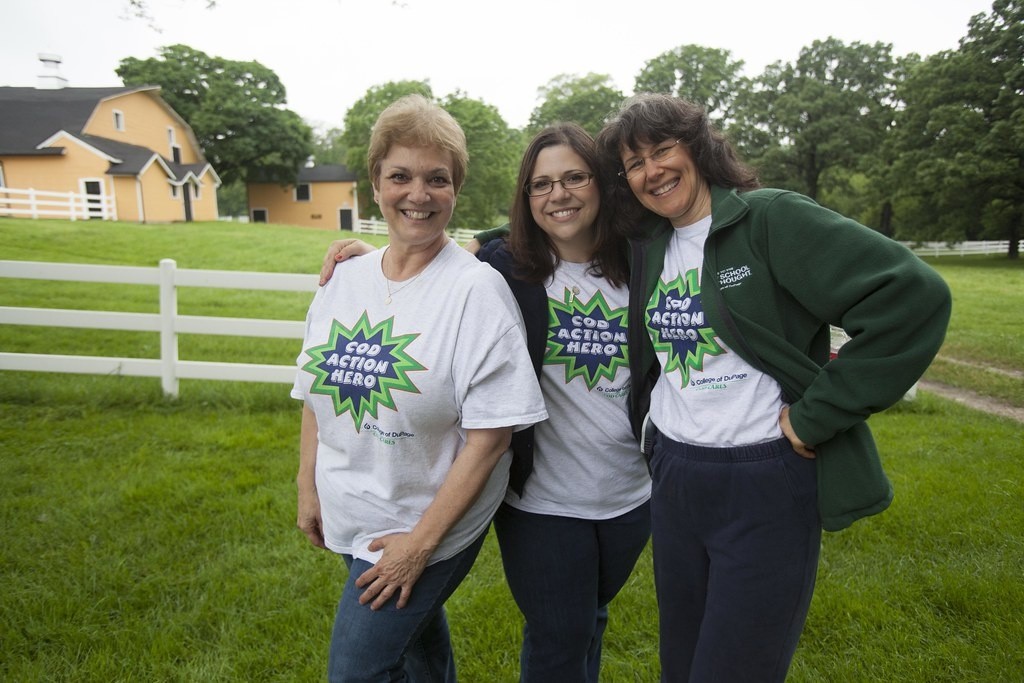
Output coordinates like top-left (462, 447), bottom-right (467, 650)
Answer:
top-left (318, 123), bottom-right (652, 683)
top-left (466, 93), bottom-right (952, 683)
top-left (290, 95), bottom-right (548, 683)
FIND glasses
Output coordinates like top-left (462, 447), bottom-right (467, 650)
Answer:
top-left (524, 171), bottom-right (595, 197)
top-left (616, 134), bottom-right (688, 180)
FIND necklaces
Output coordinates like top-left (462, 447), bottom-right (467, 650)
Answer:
top-left (557, 256), bottom-right (592, 304)
top-left (384, 248), bottom-right (429, 306)
